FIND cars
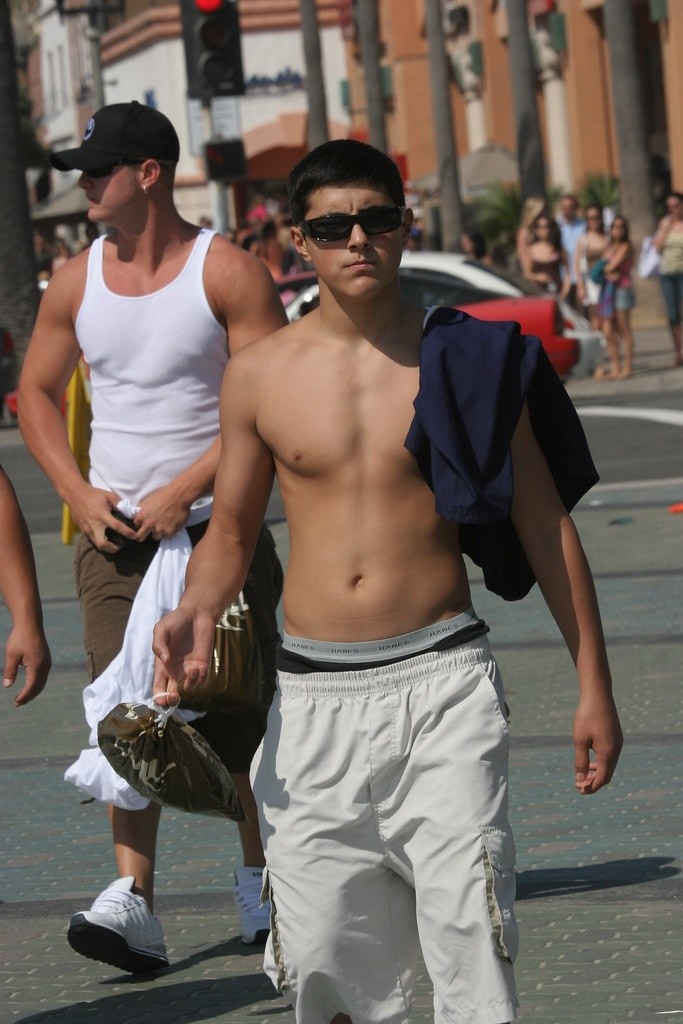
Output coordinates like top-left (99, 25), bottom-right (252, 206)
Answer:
top-left (280, 250), bottom-right (610, 381)
top-left (302, 269), bottom-right (583, 386)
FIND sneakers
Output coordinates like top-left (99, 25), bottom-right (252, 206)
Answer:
top-left (232, 864), bottom-right (271, 946)
top-left (67, 876), bottom-right (169, 973)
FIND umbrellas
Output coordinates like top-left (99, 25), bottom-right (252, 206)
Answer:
top-left (31, 177), bottom-right (94, 226)
top-left (405, 139), bottom-right (523, 192)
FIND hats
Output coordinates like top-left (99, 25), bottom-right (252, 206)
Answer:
top-left (51, 100), bottom-right (179, 171)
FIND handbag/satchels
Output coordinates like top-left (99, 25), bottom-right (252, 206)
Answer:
top-left (97, 691), bottom-right (247, 825)
top-left (638, 236), bottom-right (660, 280)
top-left (175, 584), bottom-right (271, 720)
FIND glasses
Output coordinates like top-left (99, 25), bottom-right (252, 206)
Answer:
top-left (81, 159), bottom-right (170, 179)
top-left (290, 204), bottom-right (408, 243)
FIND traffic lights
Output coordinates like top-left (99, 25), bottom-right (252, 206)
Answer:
top-left (202, 141), bottom-right (250, 184)
top-left (180, 0), bottom-right (248, 99)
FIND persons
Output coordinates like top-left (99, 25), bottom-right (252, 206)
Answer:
top-left (652, 193), bottom-right (683, 368)
top-left (0, 468), bottom-right (53, 707)
top-left (457, 189), bottom-right (634, 378)
top-left (31, 233), bottom-right (88, 291)
top-left (15, 103), bottom-right (289, 978)
top-left (196, 188), bottom-right (316, 310)
top-left (152, 140), bottom-right (623, 1023)
top-left (404, 207), bottom-right (426, 252)
